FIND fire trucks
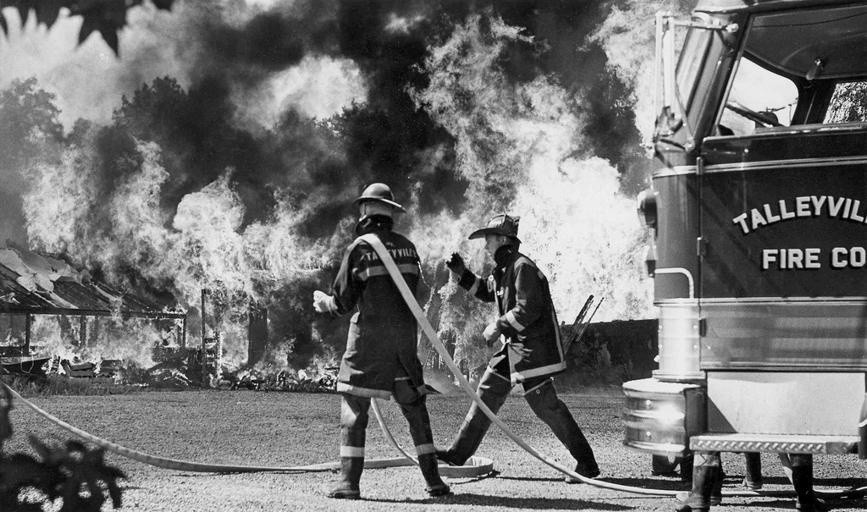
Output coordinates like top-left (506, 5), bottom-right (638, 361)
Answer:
top-left (616, 0), bottom-right (865, 479)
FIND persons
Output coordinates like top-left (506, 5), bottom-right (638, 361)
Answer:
top-left (314, 184), bottom-right (449, 495)
top-left (435, 214), bottom-right (599, 484)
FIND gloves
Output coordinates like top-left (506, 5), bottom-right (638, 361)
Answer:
top-left (482, 321), bottom-right (501, 346)
top-left (445, 251), bottom-right (467, 276)
top-left (313, 290), bottom-right (338, 314)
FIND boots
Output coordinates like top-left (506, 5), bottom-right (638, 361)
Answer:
top-left (410, 425), bottom-right (450, 496)
top-left (522, 380), bottom-right (600, 483)
top-left (433, 367), bottom-right (516, 466)
top-left (328, 427), bottom-right (366, 499)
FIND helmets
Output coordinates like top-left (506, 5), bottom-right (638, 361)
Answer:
top-left (352, 183), bottom-right (407, 213)
top-left (468, 215), bottom-right (519, 239)
